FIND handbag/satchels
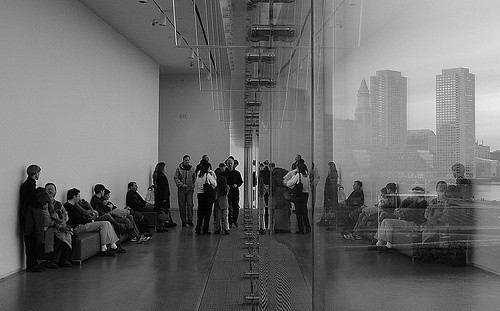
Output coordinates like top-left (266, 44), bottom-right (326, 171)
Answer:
top-left (204, 174), bottom-right (216, 198)
top-left (292, 173), bottom-right (302, 196)
top-left (159, 211), bottom-right (170, 221)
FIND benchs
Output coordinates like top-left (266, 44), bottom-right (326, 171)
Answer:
top-left (364, 221), bottom-right (423, 264)
top-left (73, 232), bottom-right (129, 266)
top-left (140, 212), bottom-right (158, 230)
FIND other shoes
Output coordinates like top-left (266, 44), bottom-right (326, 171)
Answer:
top-left (188, 221), bottom-right (193, 226)
top-left (110, 246), bottom-right (126, 253)
top-left (157, 227), bottom-right (168, 233)
top-left (100, 249), bottom-right (115, 256)
top-left (233, 221), bottom-right (238, 227)
top-left (130, 236), bottom-right (137, 241)
top-left (317, 220), bottom-right (330, 227)
top-left (225, 230), bottom-right (229, 234)
top-left (435, 256), bottom-right (448, 264)
top-left (215, 230), bottom-right (220, 234)
top-left (353, 233), bottom-right (362, 240)
top-left (182, 222), bottom-right (186, 226)
top-left (165, 222), bottom-right (176, 227)
top-left (229, 221), bottom-right (232, 228)
top-left (26, 264), bottom-right (44, 272)
top-left (379, 246), bottom-right (394, 253)
top-left (448, 260), bottom-right (465, 267)
top-left (60, 259), bottom-right (72, 267)
top-left (203, 232), bottom-right (211, 235)
top-left (140, 232), bottom-right (152, 238)
top-left (44, 261), bottom-right (59, 269)
top-left (296, 230), bottom-right (303, 233)
top-left (305, 228), bottom-right (310, 234)
top-left (423, 256), bottom-right (431, 263)
top-left (368, 244), bottom-right (384, 251)
top-left (343, 233), bottom-right (356, 240)
top-left (195, 231), bottom-right (199, 234)
top-left (137, 235), bottom-right (150, 244)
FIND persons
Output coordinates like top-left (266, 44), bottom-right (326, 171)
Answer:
top-left (317, 162), bottom-right (338, 226)
top-left (195, 154), bottom-right (212, 174)
top-left (424, 163), bottom-right (472, 267)
top-left (215, 156), bottom-right (243, 228)
top-left (63, 188), bottom-right (126, 257)
top-left (91, 184), bottom-right (153, 243)
top-left (342, 183), bottom-right (400, 242)
top-left (20, 164), bottom-right (44, 272)
top-left (153, 163), bottom-right (177, 228)
top-left (326, 181), bottom-right (364, 231)
top-left (43, 183), bottom-right (73, 269)
top-left (195, 162), bottom-right (217, 235)
top-left (197, 159), bottom-right (217, 179)
top-left (174, 155), bottom-right (197, 226)
top-left (214, 176), bottom-right (231, 235)
top-left (368, 186), bottom-right (427, 251)
top-left (260, 154), bottom-right (320, 234)
top-left (126, 182), bottom-right (168, 233)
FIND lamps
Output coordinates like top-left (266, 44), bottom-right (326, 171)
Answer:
top-left (186, 48), bottom-right (194, 61)
top-left (158, 14), bottom-right (167, 26)
top-left (205, 71), bottom-right (211, 80)
top-left (199, 61), bottom-right (204, 69)
